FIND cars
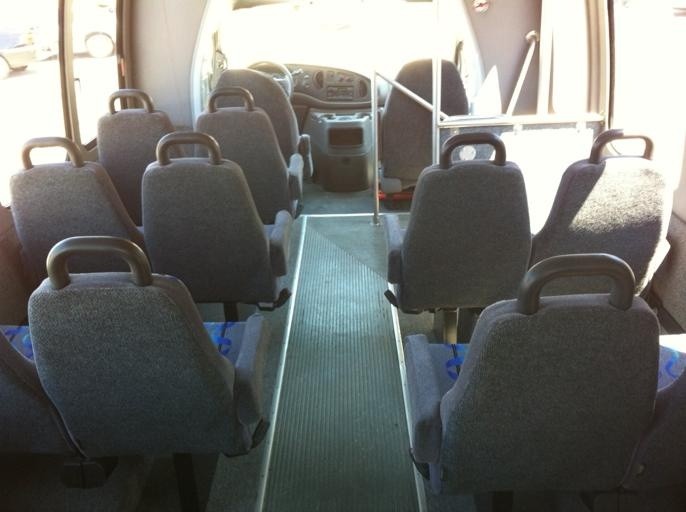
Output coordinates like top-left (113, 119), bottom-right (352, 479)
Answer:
top-left (0, 25), bottom-right (35, 79)
top-left (73, 1), bottom-right (117, 58)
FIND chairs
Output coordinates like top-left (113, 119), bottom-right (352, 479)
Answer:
top-left (0, 62), bottom-right (315, 323)
top-left (1, 236), bottom-right (271, 512)
top-left (404, 253), bottom-right (685, 511)
top-left (378, 51), bottom-right (686, 342)
